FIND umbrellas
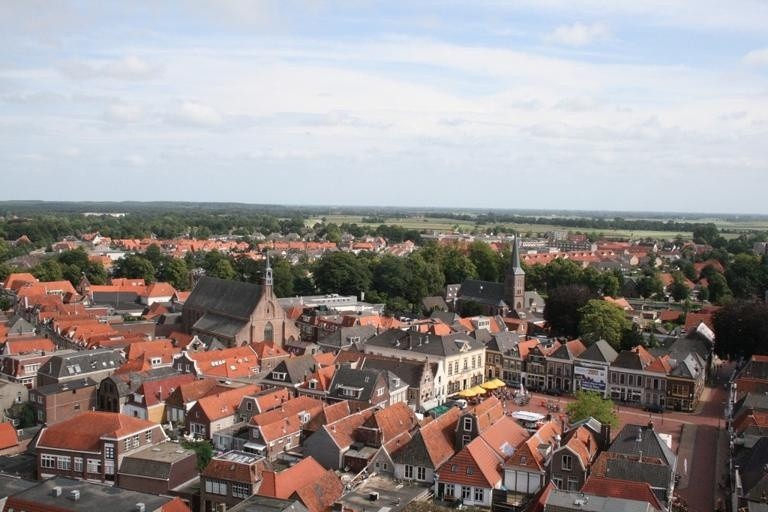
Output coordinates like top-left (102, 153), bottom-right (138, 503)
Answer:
top-left (458, 378), bottom-right (506, 401)
